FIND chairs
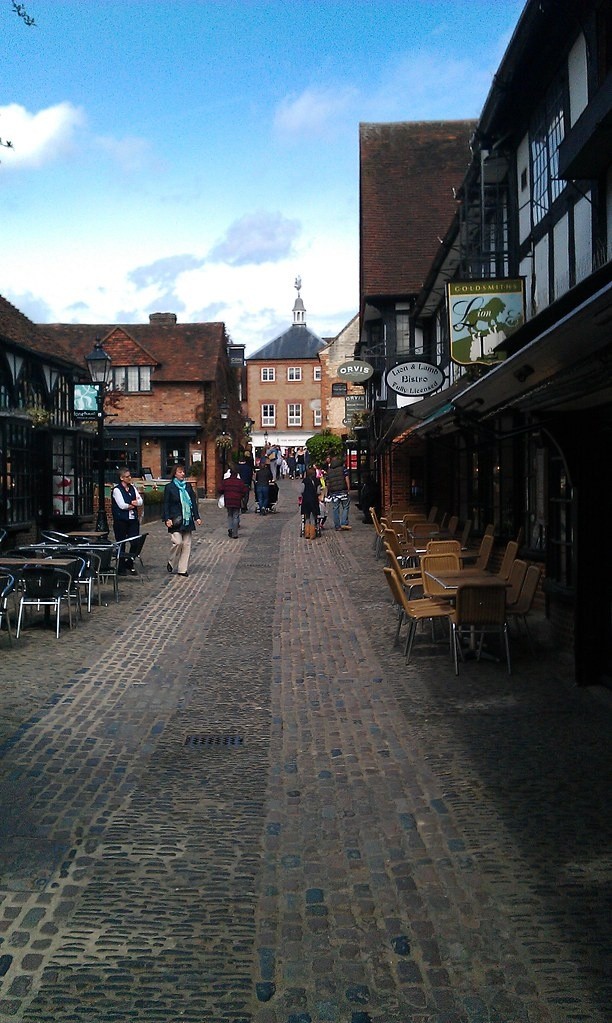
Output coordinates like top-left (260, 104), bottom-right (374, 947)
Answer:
top-left (369, 505), bottom-right (542, 677)
top-left (0, 530), bottom-right (149, 639)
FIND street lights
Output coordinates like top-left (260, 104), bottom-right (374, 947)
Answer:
top-left (83, 334), bottom-right (114, 572)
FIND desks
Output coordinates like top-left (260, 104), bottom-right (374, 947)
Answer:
top-left (423, 568), bottom-right (514, 651)
top-left (414, 546), bottom-right (466, 553)
top-left (407, 529), bottom-right (453, 545)
top-left (53, 532), bottom-right (109, 544)
top-left (0, 558), bottom-right (77, 619)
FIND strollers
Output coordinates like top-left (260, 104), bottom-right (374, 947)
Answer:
top-left (254, 481), bottom-right (279, 514)
top-left (298, 496), bottom-right (323, 538)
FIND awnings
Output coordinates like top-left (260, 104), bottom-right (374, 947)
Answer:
top-left (373, 259), bottom-right (612, 462)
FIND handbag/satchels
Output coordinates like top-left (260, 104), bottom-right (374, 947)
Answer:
top-left (165, 514), bottom-right (183, 527)
top-left (269, 452), bottom-right (275, 460)
top-left (218, 494), bottom-right (225, 509)
top-left (319, 476), bottom-right (326, 490)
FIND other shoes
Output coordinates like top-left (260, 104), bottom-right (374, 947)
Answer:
top-left (178, 572), bottom-right (188, 577)
top-left (320, 524), bottom-right (324, 530)
top-left (241, 504), bottom-right (248, 513)
top-left (167, 562), bottom-right (173, 572)
top-left (282, 473), bottom-right (304, 480)
top-left (341, 525), bottom-right (352, 530)
top-left (232, 535), bottom-right (239, 538)
top-left (126, 560), bottom-right (138, 576)
top-left (335, 526), bottom-right (341, 530)
top-left (118, 569), bottom-right (127, 576)
top-left (260, 507), bottom-right (267, 516)
top-left (227, 528), bottom-right (232, 537)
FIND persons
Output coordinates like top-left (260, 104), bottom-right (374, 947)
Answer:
top-left (255, 445), bottom-right (309, 514)
top-left (296, 464), bottom-right (326, 534)
top-left (223, 444), bottom-right (256, 538)
top-left (163, 465), bottom-right (202, 576)
top-left (111, 469), bottom-right (143, 575)
top-left (326, 458), bottom-right (352, 530)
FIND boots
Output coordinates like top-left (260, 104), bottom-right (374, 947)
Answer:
top-left (310, 525), bottom-right (317, 540)
top-left (304, 523), bottom-right (310, 539)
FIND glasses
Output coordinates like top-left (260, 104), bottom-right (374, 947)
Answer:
top-left (121, 474), bottom-right (131, 478)
